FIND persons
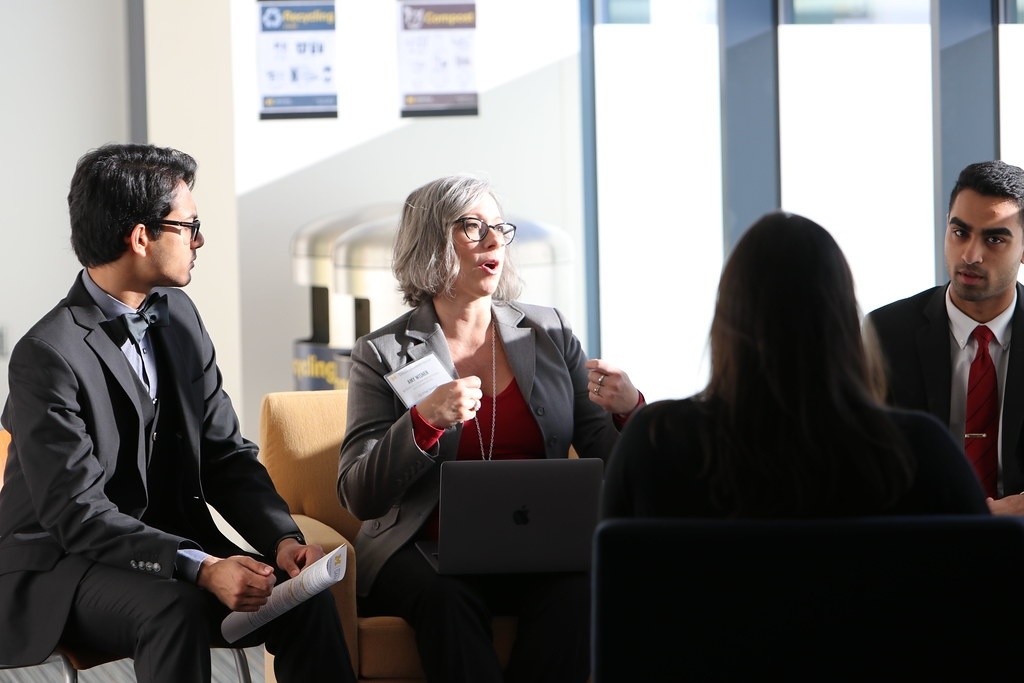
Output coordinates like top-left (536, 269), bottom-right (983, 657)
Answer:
top-left (0, 142), bottom-right (359, 683)
top-left (601, 211), bottom-right (994, 518)
top-left (336, 173), bottom-right (648, 683)
top-left (859, 161), bottom-right (1024, 519)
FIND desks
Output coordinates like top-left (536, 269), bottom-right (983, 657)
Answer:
top-left (418, 553), bottom-right (588, 683)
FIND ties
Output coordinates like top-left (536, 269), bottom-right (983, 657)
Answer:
top-left (964, 326), bottom-right (999, 501)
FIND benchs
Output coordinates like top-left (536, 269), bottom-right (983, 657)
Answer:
top-left (591, 513), bottom-right (1024, 683)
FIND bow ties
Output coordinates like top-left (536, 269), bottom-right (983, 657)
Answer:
top-left (117, 292), bottom-right (170, 345)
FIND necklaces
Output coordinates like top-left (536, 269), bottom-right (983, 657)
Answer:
top-left (452, 320), bottom-right (495, 460)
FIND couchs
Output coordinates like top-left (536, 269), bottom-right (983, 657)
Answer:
top-left (257, 389), bottom-right (580, 683)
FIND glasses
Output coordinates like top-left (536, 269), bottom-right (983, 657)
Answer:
top-left (454, 217), bottom-right (517, 245)
top-left (128, 217), bottom-right (200, 242)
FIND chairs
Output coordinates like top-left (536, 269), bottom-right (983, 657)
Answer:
top-left (0, 429), bottom-right (252, 683)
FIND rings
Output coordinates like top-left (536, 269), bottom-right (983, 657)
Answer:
top-left (470, 397), bottom-right (480, 411)
top-left (597, 375), bottom-right (605, 384)
top-left (593, 384), bottom-right (601, 396)
